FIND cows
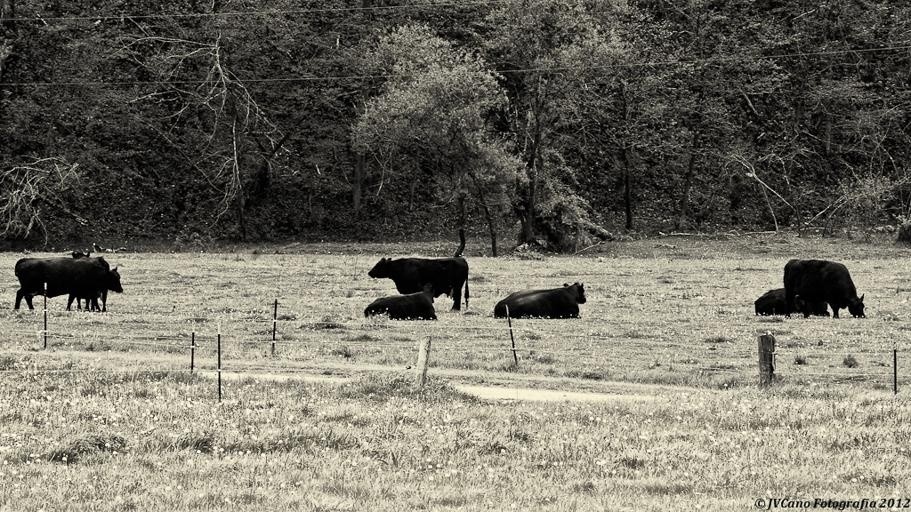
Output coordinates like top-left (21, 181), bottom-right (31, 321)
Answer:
top-left (13, 251), bottom-right (125, 315)
top-left (362, 284), bottom-right (440, 321)
top-left (782, 257), bottom-right (867, 319)
top-left (492, 280), bottom-right (587, 319)
top-left (367, 256), bottom-right (470, 311)
top-left (753, 285), bottom-right (832, 317)
